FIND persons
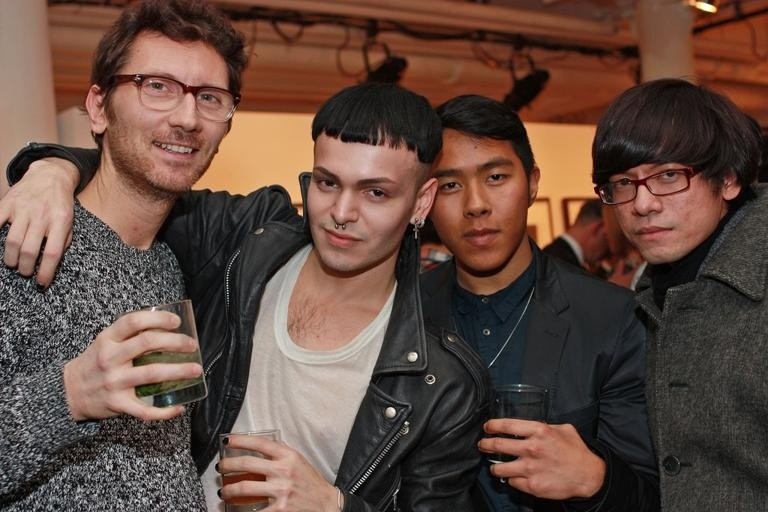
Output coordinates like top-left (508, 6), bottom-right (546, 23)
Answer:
top-left (1, 5), bottom-right (248, 512)
top-left (591, 77), bottom-right (768, 510)
top-left (421, 95), bottom-right (661, 512)
top-left (1, 80), bottom-right (492, 511)
top-left (542, 199), bottom-right (613, 275)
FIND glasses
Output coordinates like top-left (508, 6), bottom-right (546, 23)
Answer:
top-left (592, 166), bottom-right (705, 206)
top-left (107, 72), bottom-right (242, 122)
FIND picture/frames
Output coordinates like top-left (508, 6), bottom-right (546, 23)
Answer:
top-left (527, 198), bottom-right (553, 250)
top-left (563, 198), bottom-right (600, 230)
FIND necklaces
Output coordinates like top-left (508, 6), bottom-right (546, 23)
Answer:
top-left (487, 288), bottom-right (535, 372)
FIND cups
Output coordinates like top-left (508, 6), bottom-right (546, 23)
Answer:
top-left (219, 426), bottom-right (281, 512)
top-left (490, 384), bottom-right (547, 464)
top-left (122, 297), bottom-right (210, 408)
